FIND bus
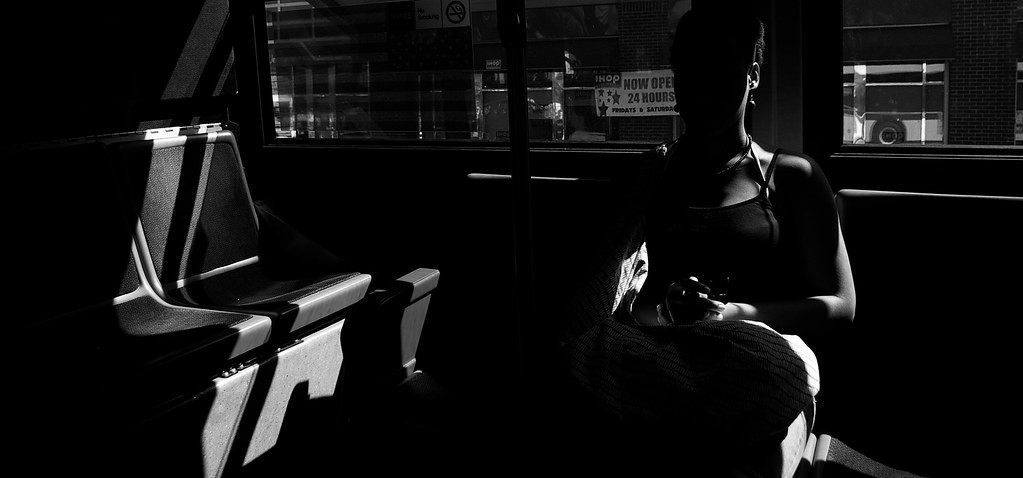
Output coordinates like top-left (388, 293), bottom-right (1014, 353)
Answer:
top-left (842, 61), bottom-right (1023, 153)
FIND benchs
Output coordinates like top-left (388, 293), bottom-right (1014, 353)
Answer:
top-left (1, 121), bottom-right (441, 478)
top-left (423, 173), bottom-right (1022, 478)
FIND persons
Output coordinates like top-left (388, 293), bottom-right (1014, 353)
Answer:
top-left (557, 5), bottom-right (855, 478)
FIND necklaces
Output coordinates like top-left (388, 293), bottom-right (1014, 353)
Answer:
top-left (679, 133), bottom-right (753, 174)
